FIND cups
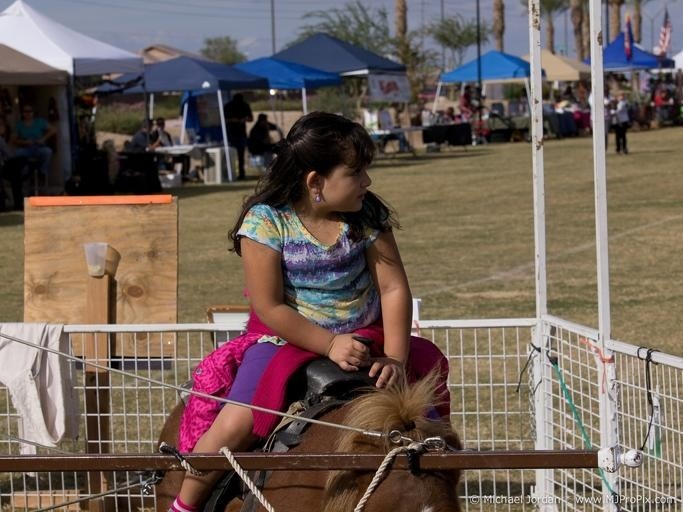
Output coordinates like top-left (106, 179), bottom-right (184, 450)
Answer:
top-left (83, 241), bottom-right (108, 279)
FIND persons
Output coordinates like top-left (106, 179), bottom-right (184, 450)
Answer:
top-left (422, 69), bottom-right (683, 154)
top-left (131, 94), bottom-right (284, 182)
top-left (0, 104), bottom-right (57, 207)
top-left (168, 112), bottom-right (413, 512)
top-left (379, 102), bottom-right (416, 152)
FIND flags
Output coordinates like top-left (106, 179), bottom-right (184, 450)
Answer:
top-left (659, 10), bottom-right (672, 57)
top-left (625, 14), bottom-right (634, 60)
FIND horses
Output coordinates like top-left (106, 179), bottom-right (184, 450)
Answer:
top-left (153, 359), bottom-right (463, 512)
